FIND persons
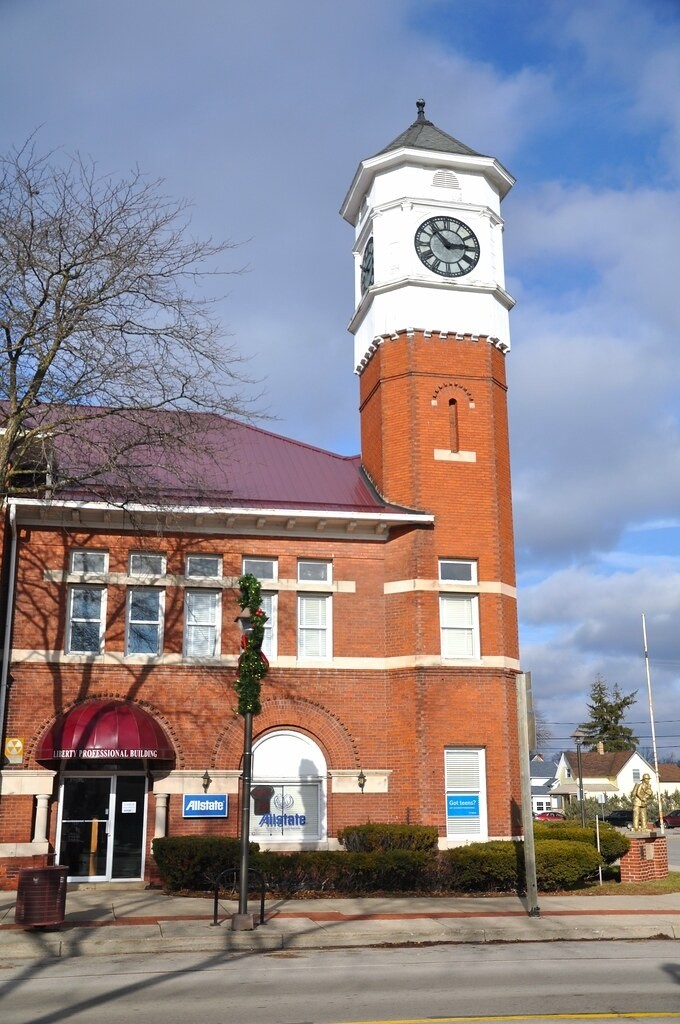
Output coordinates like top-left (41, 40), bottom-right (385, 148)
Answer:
top-left (632, 774), bottom-right (652, 831)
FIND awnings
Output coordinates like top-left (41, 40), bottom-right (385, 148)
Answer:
top-left (35, 700), bottom-right (176, 761)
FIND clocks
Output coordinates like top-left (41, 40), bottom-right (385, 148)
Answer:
top-left (360, 237), bottom-right (374, 296)
top-left (414, 216), bottom-right (480, 277)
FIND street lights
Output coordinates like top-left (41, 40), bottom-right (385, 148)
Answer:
top-left (575, 740), bottom-right (586, 828)
top-left (230, 606), bottom-right (269, 931)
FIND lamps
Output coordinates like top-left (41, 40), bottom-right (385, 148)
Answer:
top-left (358, 769), bottom-right (366, 794)
top-left (201, 770), bottom-right (211, 793)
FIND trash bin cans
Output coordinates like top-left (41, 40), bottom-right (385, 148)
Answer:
top-left (16, 865), bottom-right (70, 930)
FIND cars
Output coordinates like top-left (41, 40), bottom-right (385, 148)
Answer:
top-left (655, 810), bottom-right (680, 829)
top-left (598, 810), bottom-right (633, 829)
top-left (534, 812), bottom-right (567, 821)
